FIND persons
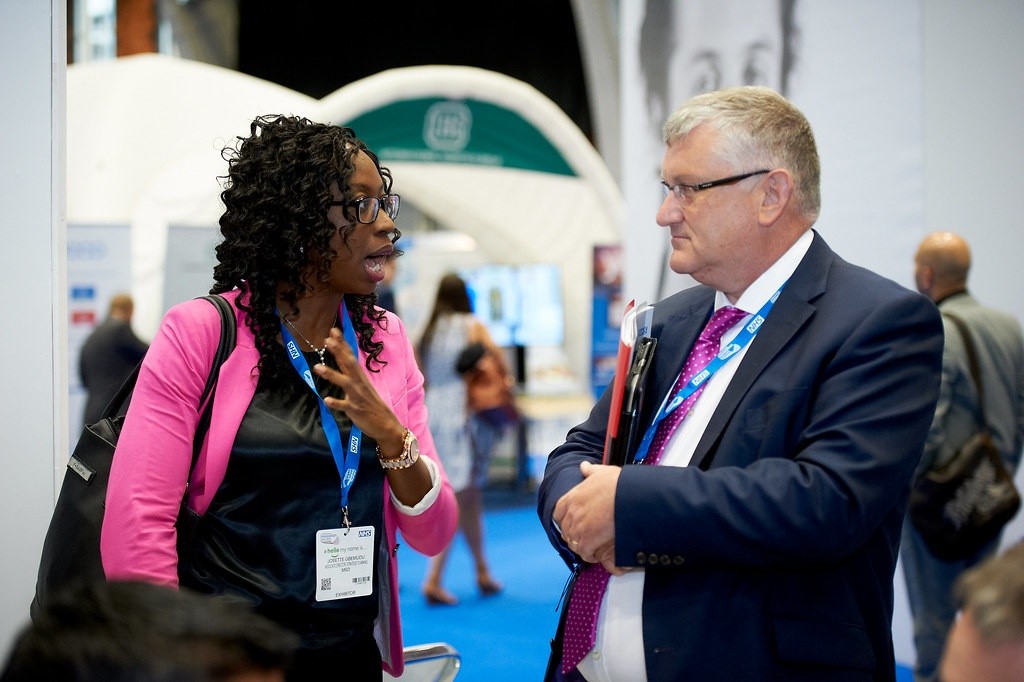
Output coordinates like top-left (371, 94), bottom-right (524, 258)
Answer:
top-left (902, 229), bottom-right (1024, 682)
top-left (77, 295), bottom-right (148, 427)
top-left (0, 577), bottom-right (294, 682)
top-left (538, 87), bottom-right (944, 682)
top-left (104, 115), bottom-right (458, 682)
top-left (415, 273), bottom-right (529, 603)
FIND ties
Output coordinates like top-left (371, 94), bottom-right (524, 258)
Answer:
top-left (561, 305), bottom-right (749, 674)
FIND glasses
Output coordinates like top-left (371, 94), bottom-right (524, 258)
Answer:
top-left (661, 169), bottom-right (773, 212)
top-left (327, 191), bottom-right (400, 225)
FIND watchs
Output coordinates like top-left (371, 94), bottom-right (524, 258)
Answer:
top-left (375, 430), bottom-right (419, 472)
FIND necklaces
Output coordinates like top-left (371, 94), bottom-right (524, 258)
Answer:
top-left (282, 312), bottom-right (337, 367)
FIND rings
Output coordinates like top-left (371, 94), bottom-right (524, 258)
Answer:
top-left (570, 540), bottom-right (578, 544)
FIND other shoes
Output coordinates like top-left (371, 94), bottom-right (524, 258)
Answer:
top-left (480, 580), bottom-right (500, 594)
top-left (425, 584), bottom-right (457, 604)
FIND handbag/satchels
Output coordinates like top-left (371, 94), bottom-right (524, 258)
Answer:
top-left (31, 288), bottom-right (280, 640)
top-left (906, 431), bottom-right (1021, 569)
top-left (457, 345), bottom-right (519, 429)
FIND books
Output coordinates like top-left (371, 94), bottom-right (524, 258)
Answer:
top-left (602, 299), bottom-right (657, 465)
